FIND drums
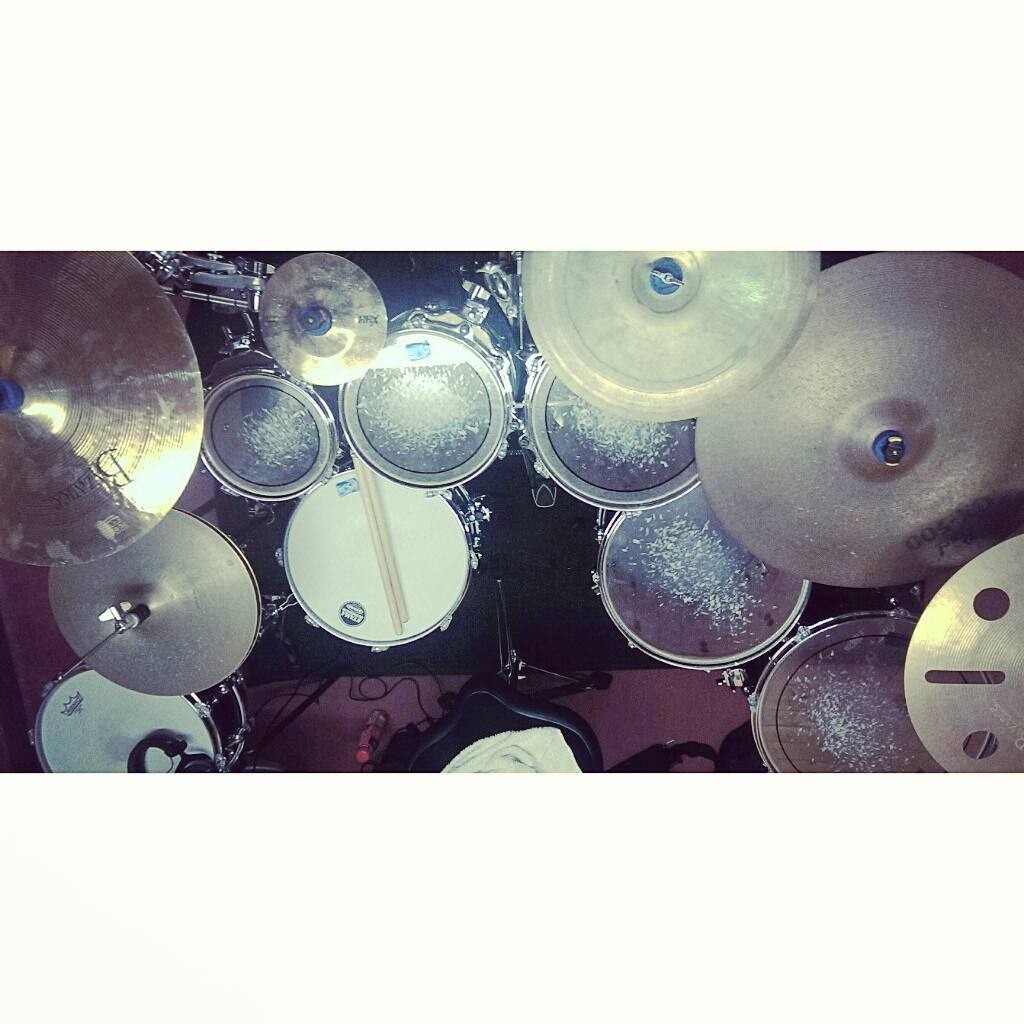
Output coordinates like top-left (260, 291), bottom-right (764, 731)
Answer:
top-left (336, 270), bottom-right (522, 493)
top-left (31, 669), bottom-right (254, 772)
top-left (523, 363), bottom-right (701, 514)
top-left (279, 453), bottom-right (487, 650)
top-left (198, 349), bottom-right (340, 503)
top-left (592, 487), bottom-right (814, 673)
top-left (743, 587), bottom-right (993, 773)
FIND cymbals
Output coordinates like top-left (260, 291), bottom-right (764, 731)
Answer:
top-left (520, 251), bottom-right (823, 425)
top-left (258, 253), bottom-right (390, 389)
top-left (45, 508), bottom-right (264, 700)
top-left (900, 531), bottom-right (1023, 773)
top-left (1, 252), bottom-right (210, 572)
top-left (692, 251), bottom-right (1023, 591)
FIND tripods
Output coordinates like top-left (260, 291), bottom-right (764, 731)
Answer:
top-left (436, 524), bottom-right (615, 710)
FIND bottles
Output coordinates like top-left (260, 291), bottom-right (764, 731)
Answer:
top-left (356, 709), bottom-right (389, 764)
